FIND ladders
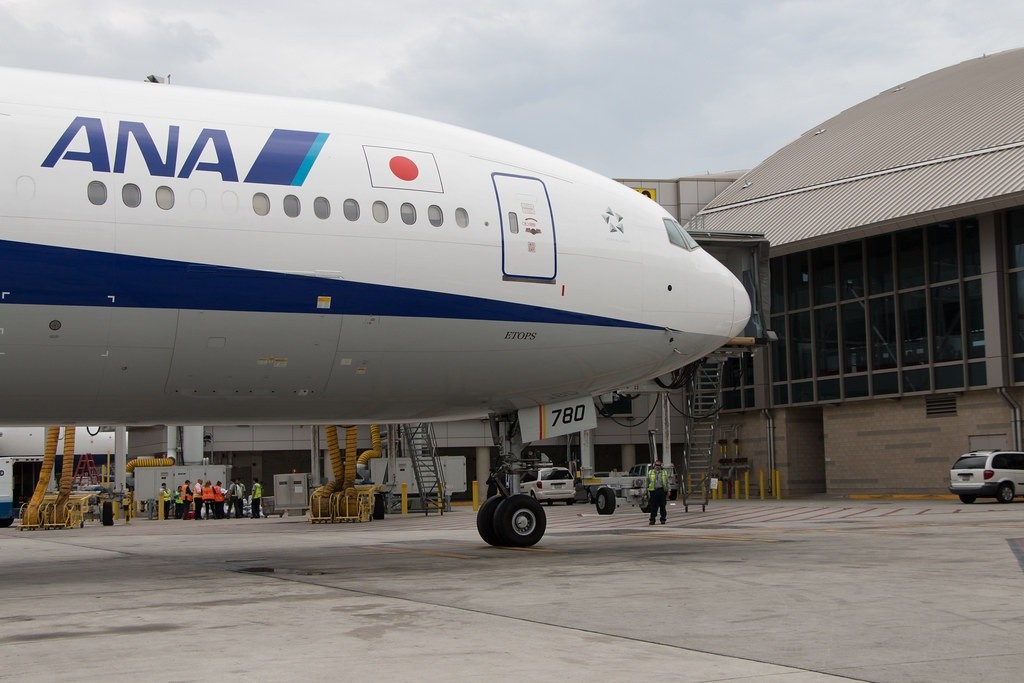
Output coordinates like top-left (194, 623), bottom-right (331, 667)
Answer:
top-left (74, 454), bottom-right (101, 486)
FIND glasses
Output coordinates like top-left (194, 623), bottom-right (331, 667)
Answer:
top-left (655, 464), bottom-right (661, 466)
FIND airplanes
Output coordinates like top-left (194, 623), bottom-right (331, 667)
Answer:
top-left (0, 65), bottom-right (753, 550)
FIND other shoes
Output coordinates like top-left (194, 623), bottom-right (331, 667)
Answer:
top-left (661, 521), bottom-right (665, 524)
top-left (649, 521), bottom-right (654, 525)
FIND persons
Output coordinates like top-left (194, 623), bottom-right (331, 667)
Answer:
top-left (485, 472), bottom-right (497, 500)
top-left (645, 461), bottom-right (671, 525)
top-left (444, 485), bottom-right (454, 513)
top-left (162, 477), bottom-right (264, 519)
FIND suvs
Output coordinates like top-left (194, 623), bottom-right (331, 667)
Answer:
top-left (947, 448), bottom-right (1024, 504)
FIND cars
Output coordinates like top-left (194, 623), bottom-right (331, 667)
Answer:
top-left (573, 476), bottom-right (598, 504)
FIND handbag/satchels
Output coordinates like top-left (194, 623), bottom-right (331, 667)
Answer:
top-left (639, 494), bottom-right (651, 513)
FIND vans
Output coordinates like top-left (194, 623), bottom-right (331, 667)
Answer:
top-left (628, 461), bottom-right (680, 500)
top-left (520, 466), bottom-right (577, 505)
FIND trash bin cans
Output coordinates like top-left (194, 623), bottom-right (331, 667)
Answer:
top-left (372, 493), bottom-right (385, 520)
